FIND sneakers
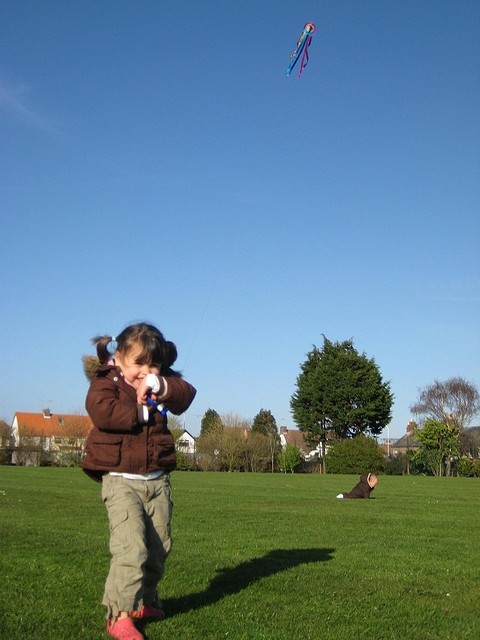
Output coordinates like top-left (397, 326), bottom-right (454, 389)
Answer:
top-left (106, 617), bottom-right (142, 639)
top-left (131, 602), bottom-right (164, 620)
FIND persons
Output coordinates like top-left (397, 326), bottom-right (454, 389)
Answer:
top-left (80, 322), bottom-right (198, 640)
top-left (336, 472), bottom-right (378, 499)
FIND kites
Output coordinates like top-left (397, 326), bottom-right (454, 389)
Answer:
top-left (285, 21), bottom-right (317, 79)
